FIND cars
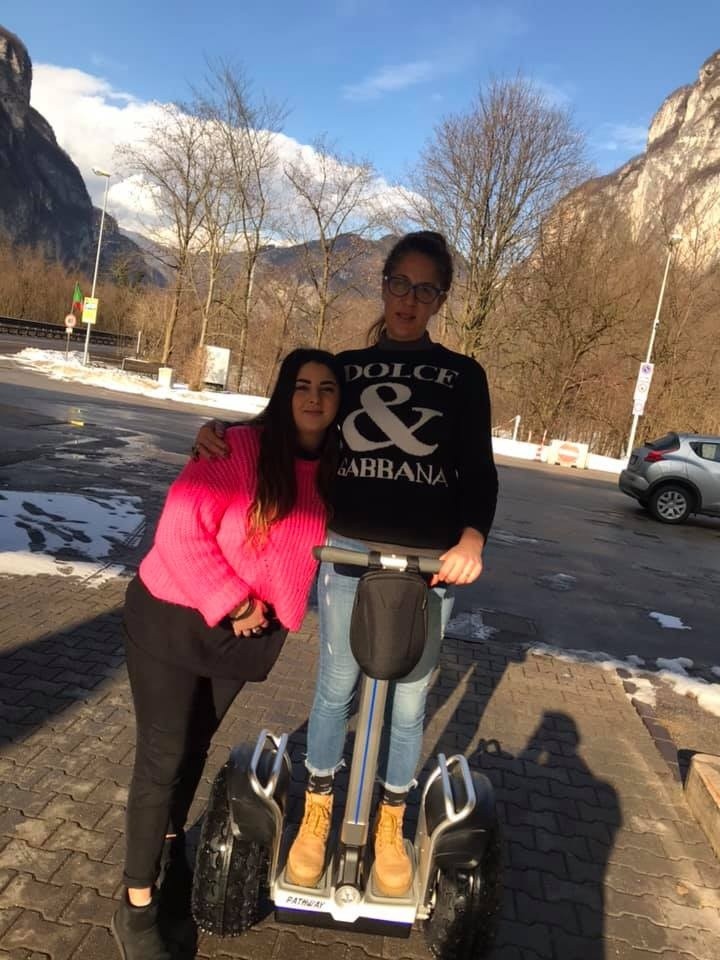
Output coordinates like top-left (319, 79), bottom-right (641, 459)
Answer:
top-left (618, 424), bottom-right (720, 524)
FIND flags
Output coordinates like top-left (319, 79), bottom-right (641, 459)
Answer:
top-left (73, 282), bottom-right (84, 312)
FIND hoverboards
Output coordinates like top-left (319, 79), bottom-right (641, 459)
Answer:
top-left (194, 548), bottom-right (505, 960)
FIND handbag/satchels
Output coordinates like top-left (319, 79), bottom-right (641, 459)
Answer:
top-left (349, 571), bottom-right (428, 681)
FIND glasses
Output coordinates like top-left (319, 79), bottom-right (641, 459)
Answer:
top-left (384, 275), bottom-right (445, 304)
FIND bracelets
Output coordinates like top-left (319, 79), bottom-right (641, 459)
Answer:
top-left (228, 598), bottom-right (253, 620)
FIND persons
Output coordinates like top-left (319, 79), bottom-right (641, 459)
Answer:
top-left (110, 347), bottom-right (345, 960)
top-left (190, 231), bottom-right (500, 895)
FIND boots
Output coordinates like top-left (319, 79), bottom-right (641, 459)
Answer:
top-left (372, 802), bottom-right (412, 896)
top-left (289, 791), bottom-right (334, 888)
top-left (159, 832), bottom-right (193, 921)
top-left (111, 884), bottom-right (174, 960)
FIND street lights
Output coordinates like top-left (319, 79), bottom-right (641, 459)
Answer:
top-left (623, 235), bottom-right (683, 474)
top-left (82, 167), bottom-right (111, 366)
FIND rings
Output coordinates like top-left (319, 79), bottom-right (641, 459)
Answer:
top-left (253, 628), bottom-right (261, 633)
